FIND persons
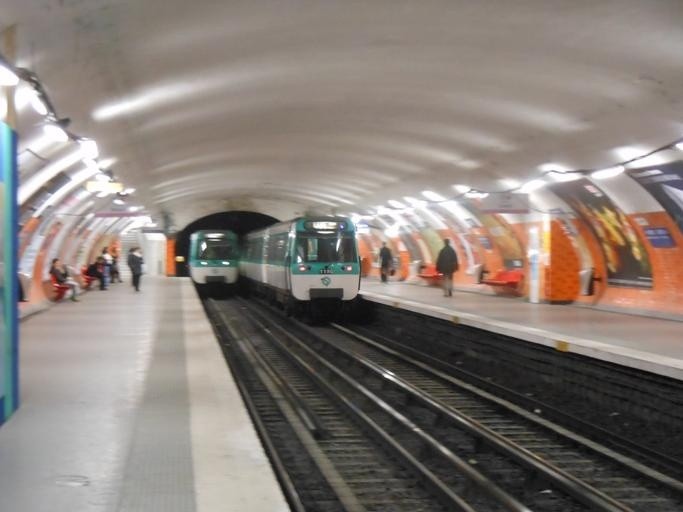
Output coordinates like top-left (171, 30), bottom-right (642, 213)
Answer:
top-left (49, 258), bottom-right (81, 301)
top-left (128, 247), bottom-right (135, 286)
top-left (436, 239), bottom-right (458, 297)
top-left (378, 241), bottom-right (394, 286)
top-left (131, 247), bottom-right (144, 291)
top-left (88, 246), bottom-right (124, 290)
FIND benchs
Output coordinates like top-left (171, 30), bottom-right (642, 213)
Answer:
top-left (479, 270), bottom-right (525, 298)
top-left (416, 263), bottom-right (444, 287)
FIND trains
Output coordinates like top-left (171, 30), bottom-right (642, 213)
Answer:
top-left (186, 228), bottom-right (240, 288)
top-left (238, 214), bottom-right (361, 320)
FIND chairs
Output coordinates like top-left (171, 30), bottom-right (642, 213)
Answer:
top-left (49, 274), bottom-right (70, 302)
top-left (80, 265), bottom-right (95, 288)
